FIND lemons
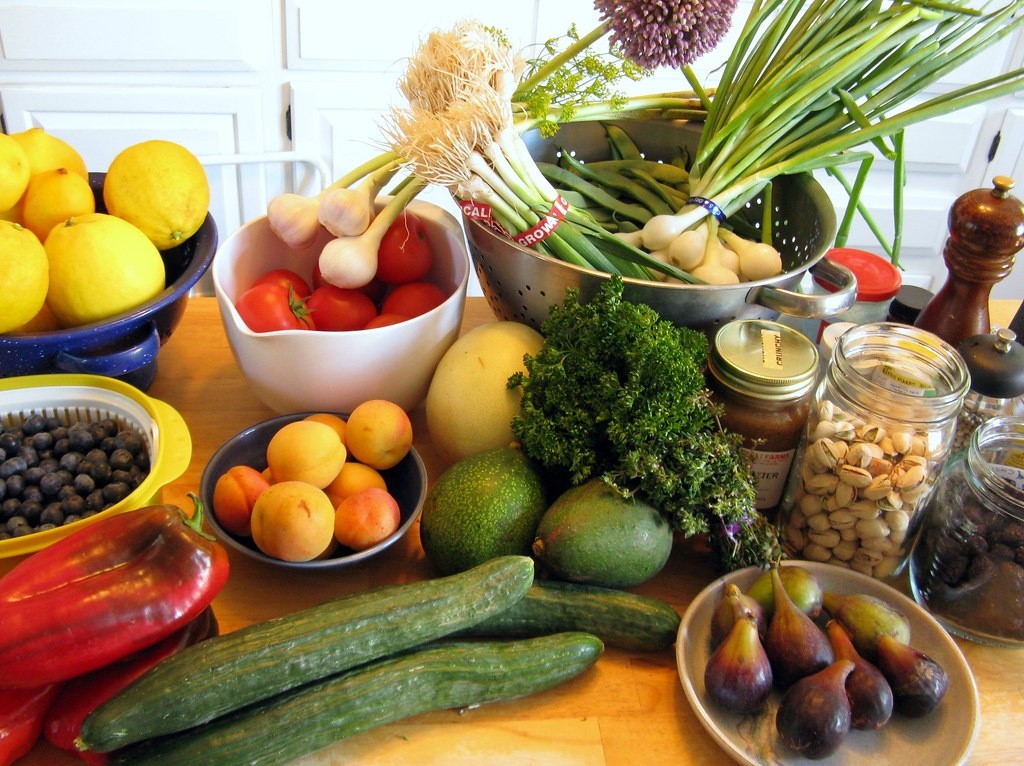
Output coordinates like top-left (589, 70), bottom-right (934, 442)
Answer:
top-left (0, 126), bottom-right (212, 338)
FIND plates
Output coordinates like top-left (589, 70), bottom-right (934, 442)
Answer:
top-left (675, 558), bottom-right (981, 766)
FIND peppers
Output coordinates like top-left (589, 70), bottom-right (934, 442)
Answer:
top-left (0, 486), bottom-right (231, 766)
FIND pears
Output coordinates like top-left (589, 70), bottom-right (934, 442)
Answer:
top-left (426, 319), bottom-right (547, 460)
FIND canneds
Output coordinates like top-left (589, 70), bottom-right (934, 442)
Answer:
top-left (706, 246), bottom-right (904, 527)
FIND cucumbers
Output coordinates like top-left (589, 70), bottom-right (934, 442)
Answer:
top-left (74, 552), bottom-right (683, 766)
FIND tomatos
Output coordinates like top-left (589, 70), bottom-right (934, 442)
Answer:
top-left (236, 212), bottom-right (449, 333)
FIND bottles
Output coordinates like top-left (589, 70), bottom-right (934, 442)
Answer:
top-left (803, 247), bottom-right (903, 380)
top-left (769, 320), bottom-right (971, 588)
top-left (948, 327), bottom-right (1023, 460)
top-left (701, 318), bottom-right (820, 526)
top-left (885, 283), bottom-right (934, 326)
top-left (910, 413), bottom-right (1023, 650)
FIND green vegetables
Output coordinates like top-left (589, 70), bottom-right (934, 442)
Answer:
top-left (503, 268), bottom-right (794, 574)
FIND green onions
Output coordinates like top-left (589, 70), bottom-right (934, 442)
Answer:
top-left (383, 0), bottom-right (1024, 284)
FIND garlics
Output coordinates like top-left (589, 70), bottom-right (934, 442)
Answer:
top-left (266, 187), bottom-right (381, 288)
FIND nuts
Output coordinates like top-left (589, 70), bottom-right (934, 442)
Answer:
top-left (778, 396), bottom-right (947, 580)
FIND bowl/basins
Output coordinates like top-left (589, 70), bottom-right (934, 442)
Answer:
top-left (199, 410), bottom-right (428, 577)
top-left (0, 372), bottom-right (192, 581)
top-left (209, 196), bottom-right (471, 422)
top-left (0, 173), bottom-right (219, 395)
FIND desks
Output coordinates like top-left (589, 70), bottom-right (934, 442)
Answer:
top-left (147, 290), bottom-right (1024, 766)
